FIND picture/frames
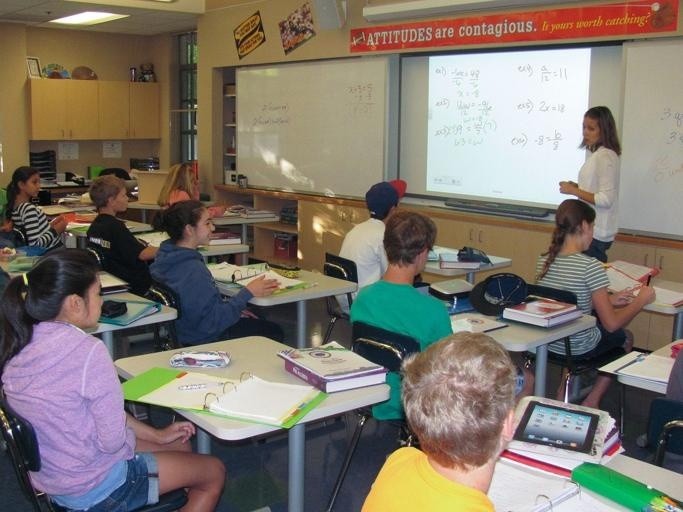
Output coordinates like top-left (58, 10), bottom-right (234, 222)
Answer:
top-left (26, 56), bottom-right (42, 78)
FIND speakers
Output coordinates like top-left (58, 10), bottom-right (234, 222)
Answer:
top-left (314, 1), bottom-right (346, 31)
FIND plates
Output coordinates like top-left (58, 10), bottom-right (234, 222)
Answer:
top-left (41, 63), bottom-right (70, 79)
top-left (71, 67), bottom-right (96, 80)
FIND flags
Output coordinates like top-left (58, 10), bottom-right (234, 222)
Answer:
top-left (208, 262), bottom-right (304, 296)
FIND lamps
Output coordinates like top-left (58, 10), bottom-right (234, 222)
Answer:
top-left (362, 0), bottom-right (623, 23)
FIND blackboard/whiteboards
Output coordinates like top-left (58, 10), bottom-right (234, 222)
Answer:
top-left (400, 40), bottom-right (624, 223)
top-left (235, 53), bottom-right (400, 201)
top-left (618, 39), bottom-right (683, 242)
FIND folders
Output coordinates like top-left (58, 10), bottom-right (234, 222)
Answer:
top-left (97, 295), bottom-right (161, 326)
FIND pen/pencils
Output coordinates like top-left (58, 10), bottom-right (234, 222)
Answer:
top-left (179, 382), bottom-right (224, 390)
top-left (647, 274), bottom-right (652, 286)
top-left (303, 282), bottom-right (320, 289)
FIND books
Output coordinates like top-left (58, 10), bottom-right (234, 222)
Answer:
top-left (428, 250), bottom-right (481, 270)
top-left (118, 368), bottom-right (328, 430)
top-left (278, 339), bottom-right (388, 395)
top-left (450, 296), bottom-right (583, 332)
top-left (208, 232), bottom-right (242, 246)
top-left (99, 271), bottom-right (128, 295)
top-left (240, 209), bottom-right (276, 218)
top-left (605, 259), bottom-right (683, 308)
top-left (497, 397), bottom-right (625, 481)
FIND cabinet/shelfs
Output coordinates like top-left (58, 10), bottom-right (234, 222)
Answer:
top-left (225, 94), bottom-right (236, 156)
top-left (28, 79), bottom-right (99, 141)
top-left (214, 185), bottom-right (298, 270)
top-left (99, 80), bottom-right (161, 141)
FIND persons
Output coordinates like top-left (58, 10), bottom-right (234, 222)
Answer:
top-left (151, 202), bottom-right (285, 343)
top-left (3, 167), bottom-right (67, 254)
top-left (157, 163), bottom-right (240, 217)
top-left (521, 196), bottom-right (656, 406)
top-left (351, 212), bottom-right (533, 421)
top-left (361, 331), bottom-right (517, 512)
top-left (85, 174), bottom-right (161, 299)
top-left (664, 342), bottom-right (683, 402)
top-left (0, 248), bottom-right (226, 512)
top-left (557, 104), bottom-right (622, 265)
top-left (333, 182), bottom-right (399, 318)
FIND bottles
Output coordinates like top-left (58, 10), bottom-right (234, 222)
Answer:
top-left (129, 68), bottom-right (136, 82)
top-left (138, 71), bottom-right (155, 83)
top-left (237, 174), bottom-right (248, 189)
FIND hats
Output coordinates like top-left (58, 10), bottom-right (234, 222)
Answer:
top-left (470, 273), bottom-right (528, 315)
top-left (366, 180), bottom-right (406, 214)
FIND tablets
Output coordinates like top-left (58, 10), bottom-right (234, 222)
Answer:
top-left (512, 401), bottom-right (599, 454)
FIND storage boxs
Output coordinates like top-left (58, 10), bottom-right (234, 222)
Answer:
top-left (131, 168), bottom-right (169, 205)
